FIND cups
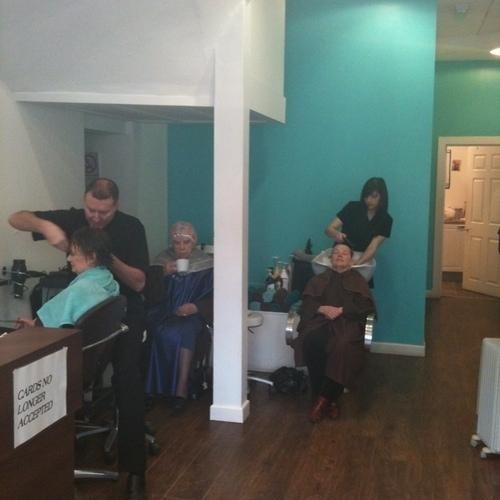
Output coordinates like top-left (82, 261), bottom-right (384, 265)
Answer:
top-left (176, 259), bottom-right (189, 276)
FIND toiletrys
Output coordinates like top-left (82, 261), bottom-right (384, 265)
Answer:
top-left (264, 257), bottom-right (289, 294)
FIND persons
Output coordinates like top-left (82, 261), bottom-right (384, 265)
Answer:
top-left (0, 226), bottom-right (120, 330)
top-left (144, 221), bottom-right (212, 418)
top-left (290, 239), bottom-right (376, 422)
top-left (8, 177), bottom-right (156, 460)
top-left (323, 177), bottom-right (394, 289)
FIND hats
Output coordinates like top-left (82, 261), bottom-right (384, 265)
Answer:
top-left (169, 221), bottom-right (197, 245)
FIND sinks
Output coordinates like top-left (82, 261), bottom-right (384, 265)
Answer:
top-left (311, 248), bottom-right (377, 283)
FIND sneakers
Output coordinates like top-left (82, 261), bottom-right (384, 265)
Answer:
top-left (145, 394), bottom-right (188, 412)
top-left (307, 394), bottom-right (335, 424)
top-left (127, 470), bottom-right (149, 500)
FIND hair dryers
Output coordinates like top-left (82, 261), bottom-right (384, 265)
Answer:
top-left (9, 257), bottom-right (44, 300)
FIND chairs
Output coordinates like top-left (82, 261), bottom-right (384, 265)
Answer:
top-left (72, 293), bottom-right (160, 482)
top-left (285, 292), bottom-right (376, 394)
